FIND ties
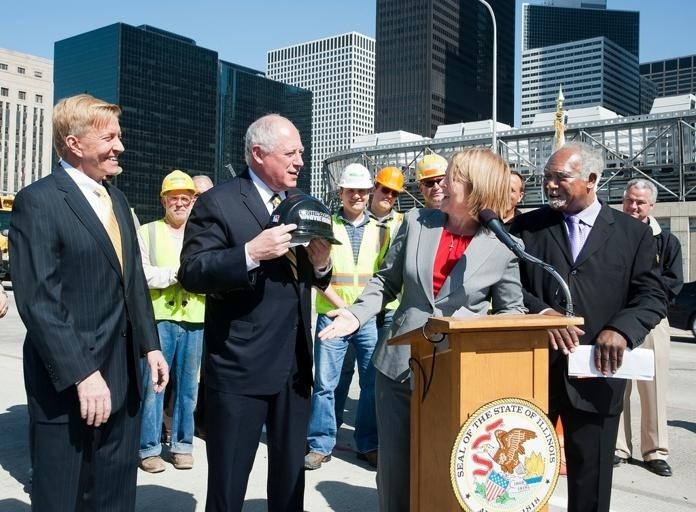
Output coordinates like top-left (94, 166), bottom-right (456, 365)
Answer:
top-left (567, 215), bottom-right (586, 265)
top-left (92, 184), bottom-right (125, 276)
top-left (269, 194), bottom-right (300, 280)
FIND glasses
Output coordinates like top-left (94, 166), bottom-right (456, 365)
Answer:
top-left (421, 178), bottom-right (444, 187)
top-left (379, 185), bottom-right (400, 198)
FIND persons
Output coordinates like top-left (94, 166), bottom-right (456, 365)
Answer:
top-left (304, 154), bottom-right (452, 471)
top-left (502, 143), bottom-right (669, 511)
top-left (135, 170), bottom-right (214, 473)
top-left (178, 114), bottom-right (332, 512)
top-left (320, 148), bottom-right (526, 512)
top-left (502, 171), bottom-right (569, 476)
top-left (612, 179), bottom-right (683, 477)
top-left (7, 94), bottom-right (170, 512)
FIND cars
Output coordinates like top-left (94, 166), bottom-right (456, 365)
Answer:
top-left (668, 280), bottom-right (696, 341)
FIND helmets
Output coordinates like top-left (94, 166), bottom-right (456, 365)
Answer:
top-left (159, 170), bottom-right (197, 198)
top-left (415, 154), bottom-right (449, 183)
top-left (375, 166), bottom-right (406, 193)
top-left (338, 162), bottom-right (374, 192)
top-left (266, 192), bottom-right (343, 246)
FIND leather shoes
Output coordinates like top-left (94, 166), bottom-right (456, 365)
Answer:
top-left (612, 454), bottom-right (631, 470)
top-left (643, 456), bottom-right (674, 477)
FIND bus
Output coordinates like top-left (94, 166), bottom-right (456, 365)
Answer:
top-left (0, 193), bottom-right (19, 285)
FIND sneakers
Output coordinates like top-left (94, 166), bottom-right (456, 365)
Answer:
top-left (304, 450), bottom-right (332, 470)
top-left (169, 452), bottom-right (195, 469)
top-left (139, 454), bottom-right (166, 474)
top-left (355, 447), bottom-right (380, 467)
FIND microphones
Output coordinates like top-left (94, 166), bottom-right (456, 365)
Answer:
top-left (478, 208), bottom-right (525, 256)
